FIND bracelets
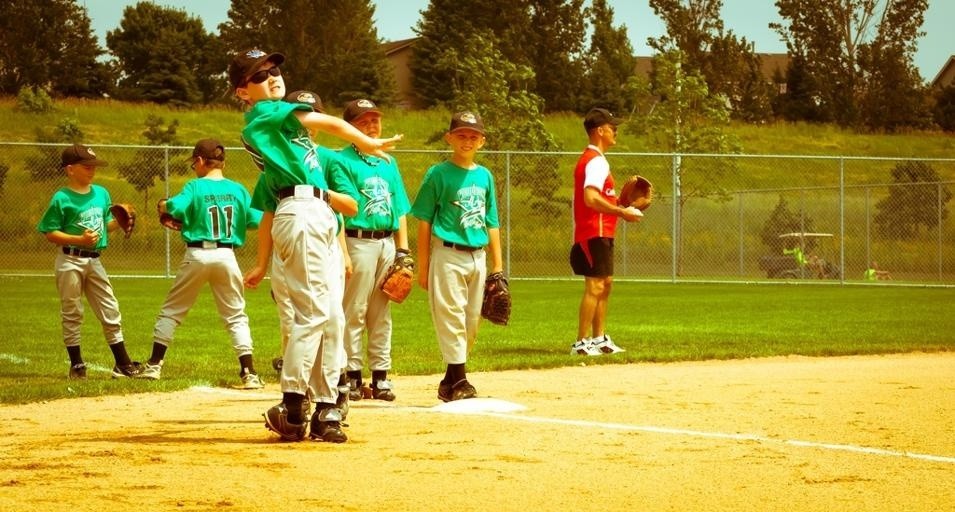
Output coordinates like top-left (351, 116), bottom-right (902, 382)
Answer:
top-left (397, 248), bottom-right (410, 254)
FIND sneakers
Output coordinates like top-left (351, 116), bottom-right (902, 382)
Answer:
top-left (112, 362), bottom-right (143, 379)
top-left (447, 382), bottom-right (477, 401)
top-left (240, 367), bottom-right (265, 389)
top-left (592, 334), bottom-right (625, 354)
top-left (438, 379), bottom-right (448, 400)
top-left (335, 386), bottom-right (348, 420)
top-left (262, 402), bottom-right (305, 442)
top-left (140, 359), bottom-right (165, 380)
top-left (350, 386), bottom-right (365, 400)
top-left (309, 409), bottom-right (346, 442)
top-left (70, 366), bottom-right (85, 380)
top-left (571, 339), bottom-right (602, 356)
top-left (372, 385), bottom-right (394, 401)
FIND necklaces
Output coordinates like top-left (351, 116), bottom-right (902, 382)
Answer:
top-left (349, 138), bottom-right (388, 167)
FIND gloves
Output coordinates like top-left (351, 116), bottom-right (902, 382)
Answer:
top-left (158, 198), bottom-right (181, 230)
top-left (379, 258), bottom-right (414, 303)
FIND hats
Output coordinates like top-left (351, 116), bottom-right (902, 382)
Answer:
top-left (584, 108), bottom-right (623, 128)
top-left (343, 100), bottom-right (384, 122)
top-left (286, 90), bottom-right (324, 114)
top-left (61, 145), bottom-right (108, 168)
top-left (448, 111), bottom-right (483, 135)
top-left (229, 47), bottom-right (286, 87)
top-left (184, 137), bottom-right (225, 160)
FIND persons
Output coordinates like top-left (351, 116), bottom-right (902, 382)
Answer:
top-left (35, 144), bottom-right (138, 380)
top-left (243, 90), bottom-right (356, 420)
top-left (784, 241), bottom-right (818, 265)
top-left (134, 138), bottom-right (266, 386)
top-left (862, 260), bottom-right (891, 280)
top-left (228, 47), bottom-right (404, 443)
top-left (411, 109), bottom-right (512, 402)
top-left (569, 107), bottom-right (644, 356)
top-left (336, 99), bottom-right (414, 400)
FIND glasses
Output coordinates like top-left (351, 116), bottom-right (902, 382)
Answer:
top-left (611, 126), bottom-right (618, 132)
top-left (191, 160), bottom-right (197, 170)
top-left (240, 66), bottom-right (281, 84)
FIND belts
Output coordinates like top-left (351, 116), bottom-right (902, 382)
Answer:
top-left (187, 240), bottom-right (233, 248)
top-left (63, 247), bottom-right (100, 258)
top-left (279, 186), bottom-right (331, 205)
top-left (345, 229), bottom-right (393, 239)
top-left (443, 241), bottom-right (483, 252)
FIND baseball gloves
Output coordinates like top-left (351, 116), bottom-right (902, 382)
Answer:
top-left (380, 250), bottom-right (416, 304)
top-left (158, 198), bottom-right (185, 234)
